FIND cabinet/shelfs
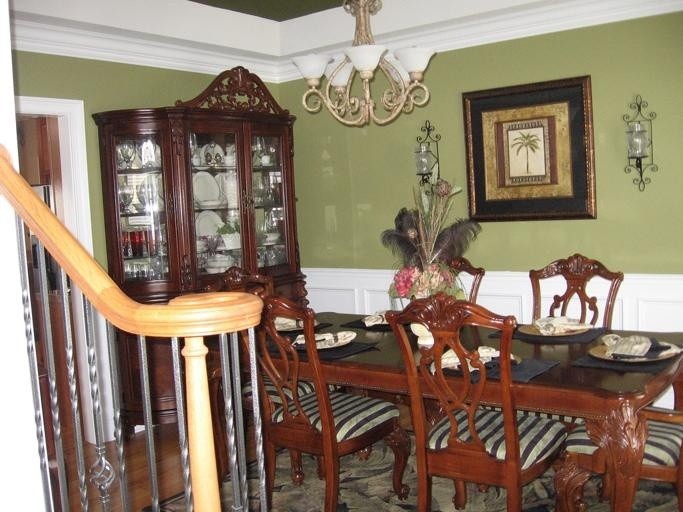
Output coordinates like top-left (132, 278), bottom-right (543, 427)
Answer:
top-left (91, 107), bottom-right (309, 426)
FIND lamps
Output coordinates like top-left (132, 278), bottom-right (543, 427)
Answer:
top-left (623, 94), bottom-right (658, 192)
top-left (414, 119), bottom-right (441, 188)
top-left (292, 0), bottom-right (436, 128)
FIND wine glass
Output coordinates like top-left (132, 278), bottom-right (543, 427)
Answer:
top-left (253, 135), bottom-right (282, 267)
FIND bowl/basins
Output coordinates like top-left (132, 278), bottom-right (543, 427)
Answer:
top-left (205, 257), bottom-right (235, 275)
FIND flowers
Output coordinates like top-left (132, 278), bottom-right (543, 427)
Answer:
top-left (387, 262), bottom-right (470, 303)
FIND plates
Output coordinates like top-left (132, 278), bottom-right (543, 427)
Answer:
top-left (191, 170), bottom-right (220, 200)
top-left (517, 324), bottom-right (589, 336)
top-left (316, 330), bottom-right (357, 352)
top-left (277, 320), bottom-right (322, 334)
top-left (588, 341), bottom-right (683, 363)
top-left (201, 142), bottom-right (224, 164)
top-left (195, 210), bottom-right (224, 237)
top-left (360, 316), bottom-right (390, 325)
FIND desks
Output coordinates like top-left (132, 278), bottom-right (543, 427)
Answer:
top-left (252, 310), bottom-right (683, 511)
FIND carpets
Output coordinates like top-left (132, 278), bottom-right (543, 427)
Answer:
top-left (142, 431), bottom-right (683, 512)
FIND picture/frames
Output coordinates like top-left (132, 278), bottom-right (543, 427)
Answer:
top-left (462, 75), bottom-right (597, 222)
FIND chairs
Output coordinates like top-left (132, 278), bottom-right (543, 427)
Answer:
top-left (384, 292), bottom-right (567, 510)
top-left (389, 257), bottom-right (488, 457)
top-left (237, 267), bottom-right (411, 512)
top-left (528, 252), bottom-right (624, 423)
top-left (203, 265), bottom-right (340, 486)
top-left (566, 407), bottom-right (683, 512)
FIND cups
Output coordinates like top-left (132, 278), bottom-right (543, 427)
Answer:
top-left (116, 139), bottom-right (158, 279)
top-left (196, 241), bottom-right (203, 252)
